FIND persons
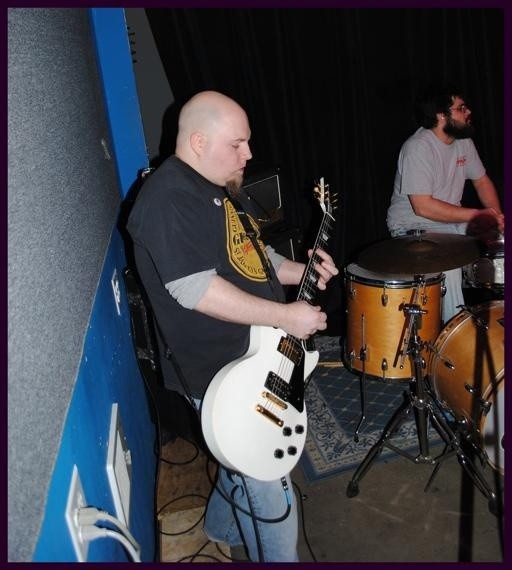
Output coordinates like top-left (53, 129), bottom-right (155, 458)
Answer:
top-left (383, 78), bottom-right (505, 332)
top-left (129, 89), bottom-right (342, 564)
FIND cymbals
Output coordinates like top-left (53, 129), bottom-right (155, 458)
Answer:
top-left (355, 232), bottom-right (487, 274)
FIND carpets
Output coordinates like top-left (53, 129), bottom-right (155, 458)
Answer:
top-left (298, 333), bottom-right (458, 484)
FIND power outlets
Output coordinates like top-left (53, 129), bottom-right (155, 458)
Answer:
top-left (64, 464), bottom-right (92, 562)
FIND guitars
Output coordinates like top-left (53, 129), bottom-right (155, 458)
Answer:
top-left (198, 175), bottom-right (339, 482)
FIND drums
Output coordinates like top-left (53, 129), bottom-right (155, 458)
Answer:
top-left (341, 263), bottom-right (447, 384)
top-left (427, 300), bottom-right (505, 477)
top-left (463, 230), bottom-right (505, 299)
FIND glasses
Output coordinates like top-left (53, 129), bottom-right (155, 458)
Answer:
top-left (449, 105), bottom-right (470, 113)
